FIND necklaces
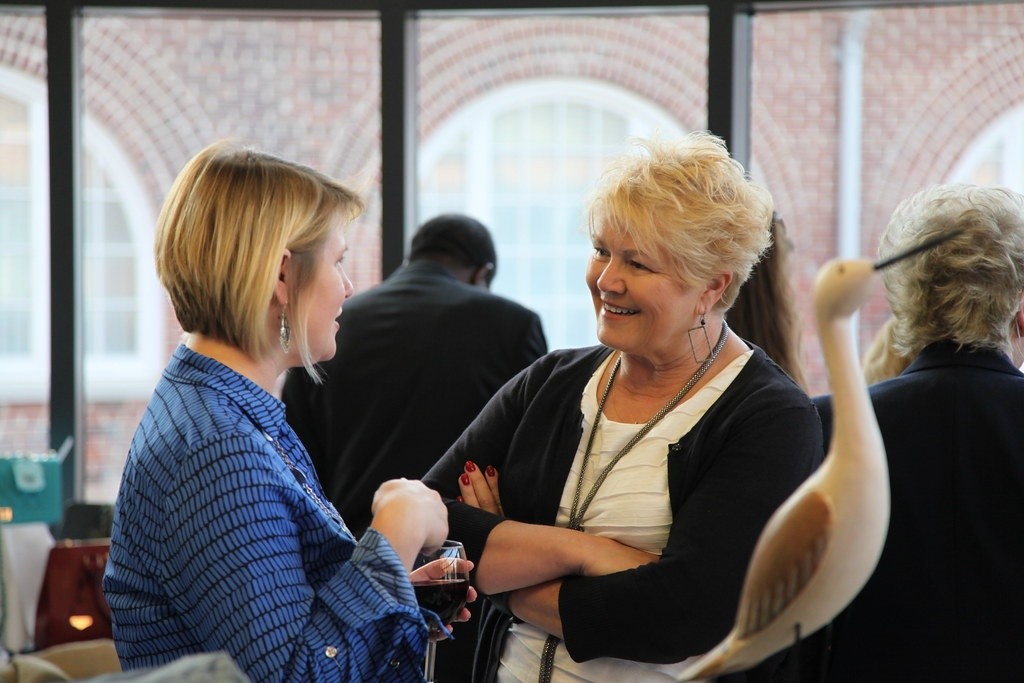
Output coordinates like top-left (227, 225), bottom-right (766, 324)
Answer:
top-left (538, 317), bottom-right (729, 683)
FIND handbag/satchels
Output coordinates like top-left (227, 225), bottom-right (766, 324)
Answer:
top-left (34, 544), bottom-right (112, 652)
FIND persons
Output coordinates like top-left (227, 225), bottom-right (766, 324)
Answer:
top-left (281, 213), bottom-right (549, 683)
top-left (100, 138), bottom-right (477, 683)
top-left (811, 181), bottom-right (1023, 682)
top-left (418, 127), bottom-right (825, 683)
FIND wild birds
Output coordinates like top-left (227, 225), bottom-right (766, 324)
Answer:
top-left (673, 229), bottom-right (962, 683)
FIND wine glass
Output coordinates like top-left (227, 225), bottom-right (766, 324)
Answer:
top-left (410, 541), bottom-right (469, 683)
